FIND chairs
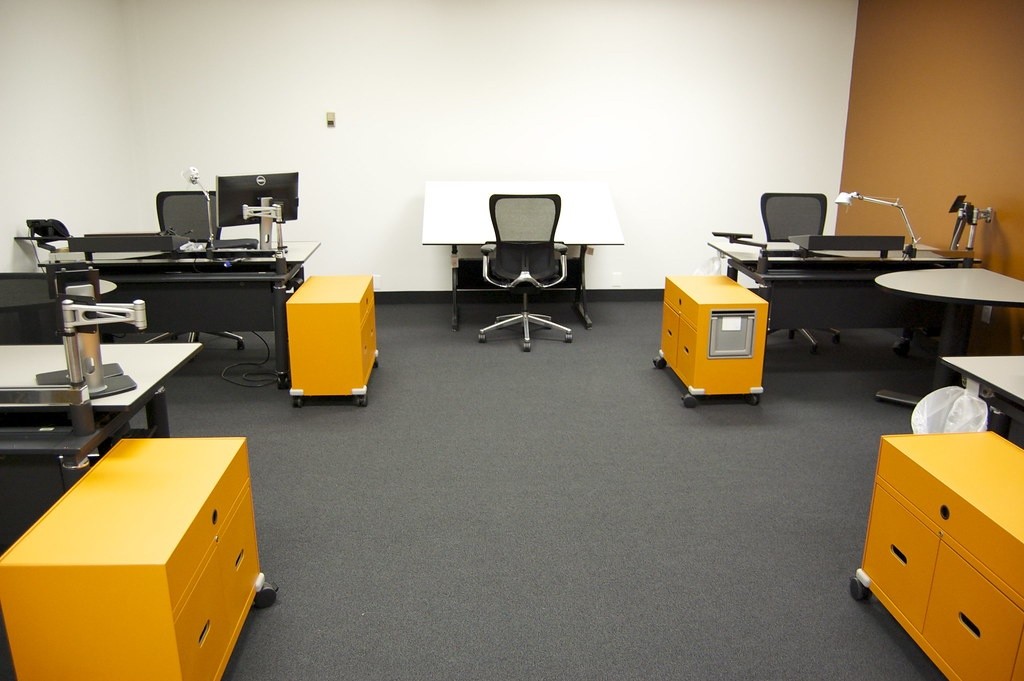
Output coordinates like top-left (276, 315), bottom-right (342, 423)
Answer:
top-left (142, 191), bottom-right (245, 362)
top-left (760, 192), bottom-right (842, 353)
top-left (478, 193), bottom-right (573, 352)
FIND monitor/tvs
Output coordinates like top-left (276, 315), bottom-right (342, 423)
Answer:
top-left (216, 172), bottom-right (298, 228)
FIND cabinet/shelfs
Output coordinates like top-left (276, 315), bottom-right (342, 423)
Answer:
top-left (285, 275), bottom-right (378, 407)
top-left (0, 437), bottom-right (279, 680)
top-left (850, 431), bottom-right (1024, 681)
top-left (652, 273), bottom-right (769, 408)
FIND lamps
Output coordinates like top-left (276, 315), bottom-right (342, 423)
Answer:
top-left (835, 190), bottom-right (921, 249)
top-left (180, 166), bottom-right (215, 251)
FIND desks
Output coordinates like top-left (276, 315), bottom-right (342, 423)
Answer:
top-left (38, 239), bottom-right (322, 390)
top-left (707, 240), bottom-right (982, 358)
top-left (939, 356), bottom-right (1024, 439)
top-left (422, 234), bottom-right (625, 332)
top-left (0, 343), bottom-right (205, 495)
top-left (874, 268), bottom-right (1024, 407)
